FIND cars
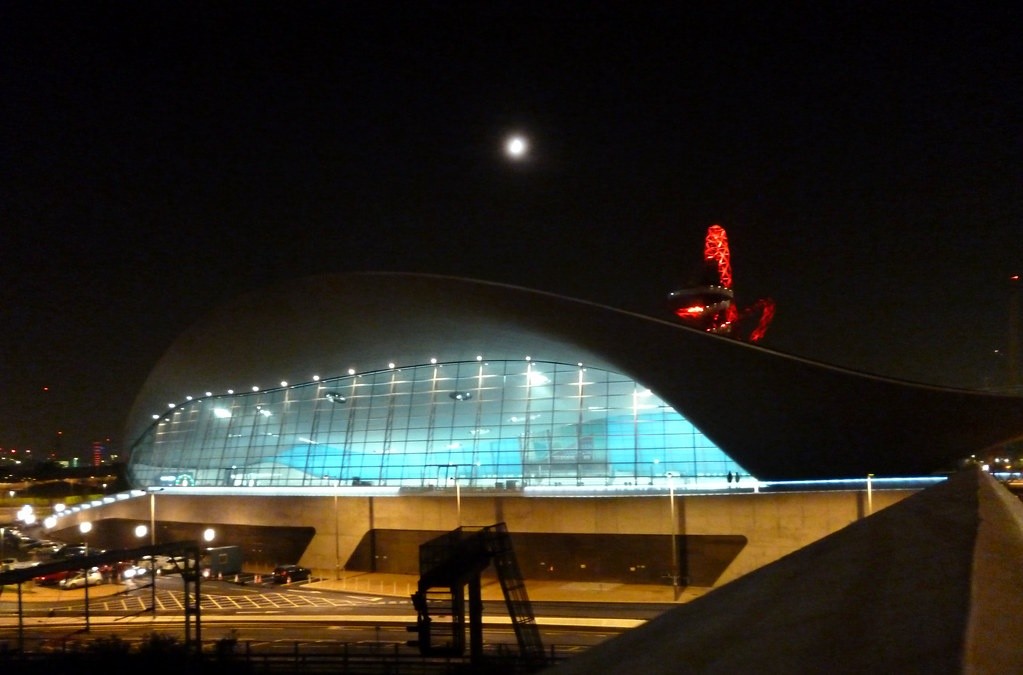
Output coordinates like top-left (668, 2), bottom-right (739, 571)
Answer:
top-left (32, 569), bottom-right (71, 585)
top-left (96, 556), bottom-right (169, 578)
top-left (271, 565), bottom-right (311, 584)
top-left (19, 538), bottom-right (90, 559)
top-left (59, 570), bottom-right (103, 590)
top-left (162, 557), bottom-right (195, 573)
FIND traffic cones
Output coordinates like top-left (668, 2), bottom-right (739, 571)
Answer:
top-left (234, 574), bottom-right (242, 583)
top-left (286, 577), bottom-right (292, 586)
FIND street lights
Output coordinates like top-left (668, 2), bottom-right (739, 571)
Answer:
top-left (81, 523), bottom-right (92, 632)
top-left (324, 475), bottom-right (340, 581)
top-left (142, 489), bottom-right (164, 618)
top-left (450, 478), bottom-right (461, 528)
top-left (667, 473), bottom-right (678, 602)
top-left (867, 471), bottom-right (874, 514)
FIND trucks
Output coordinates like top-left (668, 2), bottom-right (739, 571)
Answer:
top-left (194, 546), bottom-right (241, 577)
